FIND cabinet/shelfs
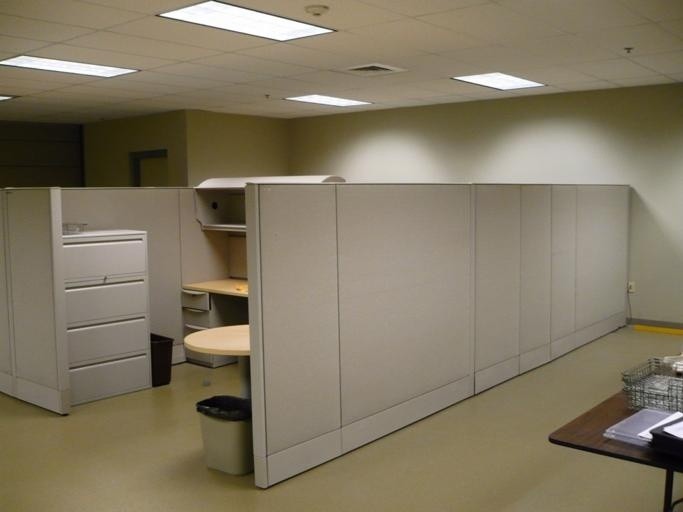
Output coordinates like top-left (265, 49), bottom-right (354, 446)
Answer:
top-left (61, 229), bottom-right (152, 407)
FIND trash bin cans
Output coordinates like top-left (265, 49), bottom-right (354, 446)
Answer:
top-left (196, 395), bottom-right (253, 475)
top-left (150, 333), bottom-right (176, 387)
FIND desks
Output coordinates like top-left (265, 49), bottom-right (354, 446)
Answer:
top-left (180, 278), bottom-right (250, 400)
top-left (547, 388), bottom-right (683, 512)
